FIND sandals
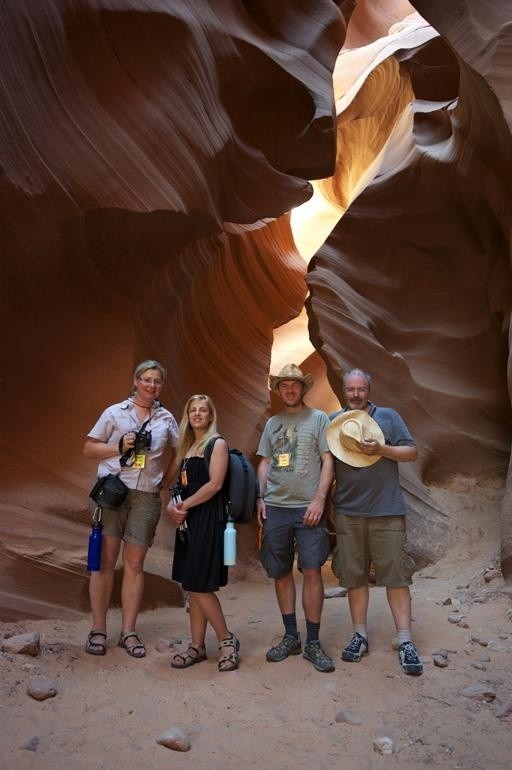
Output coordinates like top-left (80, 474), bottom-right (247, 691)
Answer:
top-left (84, 630), bottom-right (106, 655)
top-left (117, 630), bottom-right (146, 658)
top-left (171, 642), bottom-right (208, 668)
top-left (216, 632), bottom-right (241, 672)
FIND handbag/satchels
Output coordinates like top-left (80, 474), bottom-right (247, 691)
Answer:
top-left (88, 474), bottom-right (129, 511)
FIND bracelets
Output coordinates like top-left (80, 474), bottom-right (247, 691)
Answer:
top-left (256, 496), bottom-right (265, 499)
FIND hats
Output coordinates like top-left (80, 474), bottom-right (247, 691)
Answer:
top-left (270, 364), bottom-right (313, 395)
top-left (326, 409), bottom-right (386, 468)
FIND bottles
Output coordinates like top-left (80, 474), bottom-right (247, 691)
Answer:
top-left (224, 517), bottom-right (238, 566)
top-left (87, 522), bottom-right (103, 572)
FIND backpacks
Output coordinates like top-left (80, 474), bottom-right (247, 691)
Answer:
top-left (204, 436), bottom-right (256, 523)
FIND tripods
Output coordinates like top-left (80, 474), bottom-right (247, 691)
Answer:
top-left (166, 484), bottom-right (193, 544)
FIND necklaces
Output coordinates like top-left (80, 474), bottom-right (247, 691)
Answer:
top-left (133, 401), bottom-right (152, 426)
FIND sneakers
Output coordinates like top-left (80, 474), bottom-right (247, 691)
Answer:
top-left (300, 639), bottom-right (336, 672)
top-left (264, 632), bottom-right (304, 662)
top-left (396, 640), bottom-right (423, 675)
top-left (341, 631), bottom-right (370, 662)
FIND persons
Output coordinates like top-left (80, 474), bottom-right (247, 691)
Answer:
top-left (164, 393), bottom-right (241, 672)
top-left (255, 363), bottom-right (337, 672)
top-left (81, 359), bottom-right (181, 658)
top-left (328, 367), bottom-right (425, 676)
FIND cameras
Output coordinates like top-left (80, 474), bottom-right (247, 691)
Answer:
top-left (120, 432), bottom-right (153, 451)
